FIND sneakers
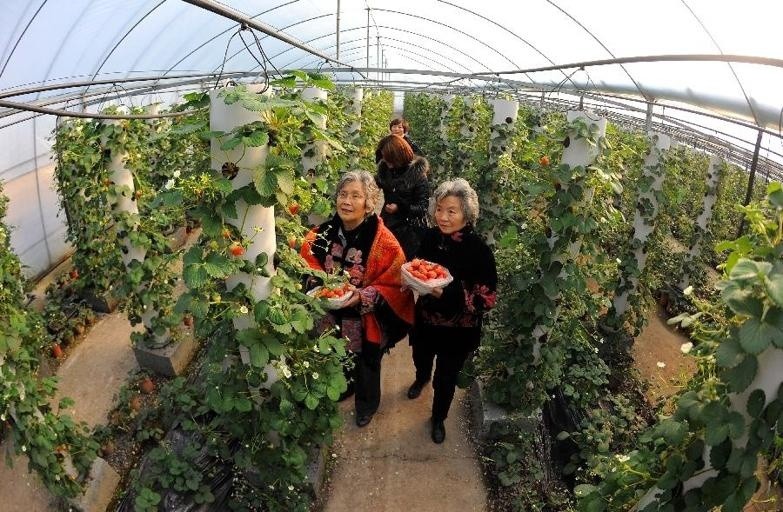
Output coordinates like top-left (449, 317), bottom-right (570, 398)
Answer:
top-left (407, 377), bottom-right (432, 399)
top-left (431, 416), bottom-right (445, 443)
top-left (333, 381), bottom-right (357, 402)
top-left (357, 416), bottom-right (371, 426)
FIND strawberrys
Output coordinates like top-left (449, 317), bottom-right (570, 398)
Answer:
top-left (316, 280), bottom-right (351, 299)
top-left (405, 257), bottom-right (450, 283)
top-left (540, 155), bottom-right (549, 166)
top-left (221, 203), bottom-right (300, 256)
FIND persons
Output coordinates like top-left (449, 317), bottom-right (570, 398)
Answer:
top-left (404, 177), bottom-right (499, 444)
top-left (294, 168), bottom-right (415, 427)
top-left (374, 133), bottom-right (430, 262)
top-left (374, 118), bottom-right (424, 165)
